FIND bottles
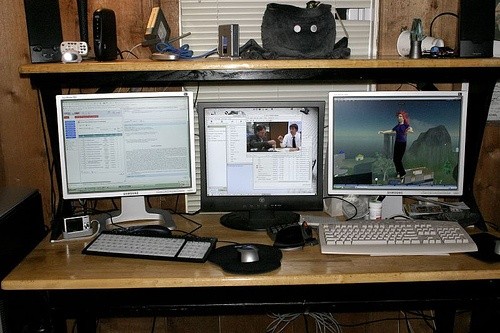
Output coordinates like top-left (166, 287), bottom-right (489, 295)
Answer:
top-left (369, 201), bottom-right (382, 221)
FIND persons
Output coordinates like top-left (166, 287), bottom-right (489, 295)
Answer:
top-left (249, 125), bottom-right (276, 152)
top-left (277, 124), bottom-right (301, 148)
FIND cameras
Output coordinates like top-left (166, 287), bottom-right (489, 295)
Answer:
top-left (63, 215), bottom-right (91, 232)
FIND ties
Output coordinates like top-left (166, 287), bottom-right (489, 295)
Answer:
top-left (292, 136), bottom-right (296, 148)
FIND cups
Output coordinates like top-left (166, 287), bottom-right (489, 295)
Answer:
top-left (396, 30), bottom-right (446, 57)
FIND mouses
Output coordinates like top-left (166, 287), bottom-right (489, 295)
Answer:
top-left (489, 240), bottom-right (500, 258)
top-left (232, 242), bottom-right (262, 269)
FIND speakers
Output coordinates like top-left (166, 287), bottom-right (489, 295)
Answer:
top-left (23, 1), bottom-right (65, 63)
top-left (457, 1), bottom-right (493, 57)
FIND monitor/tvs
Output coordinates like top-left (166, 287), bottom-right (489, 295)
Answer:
top-left (197, 100), bottom-right (324, 230)
top-left (53, 91), bottom-right (197, 231)
top-left (328, 89), bottom-right (464, 219)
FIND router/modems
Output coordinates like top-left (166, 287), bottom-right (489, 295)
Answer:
top-left (144, 7), bottom-right (191, 53)
top-left (93, 9), bottom-right (120, 59)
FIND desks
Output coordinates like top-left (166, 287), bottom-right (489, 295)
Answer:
top-left (1, 57), bottom-right (500, 333)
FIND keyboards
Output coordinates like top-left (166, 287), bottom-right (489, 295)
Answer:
top-left (82, 232), bottom-right (218, 262)
top-left (318, 220), bottom-right (478, 256)
